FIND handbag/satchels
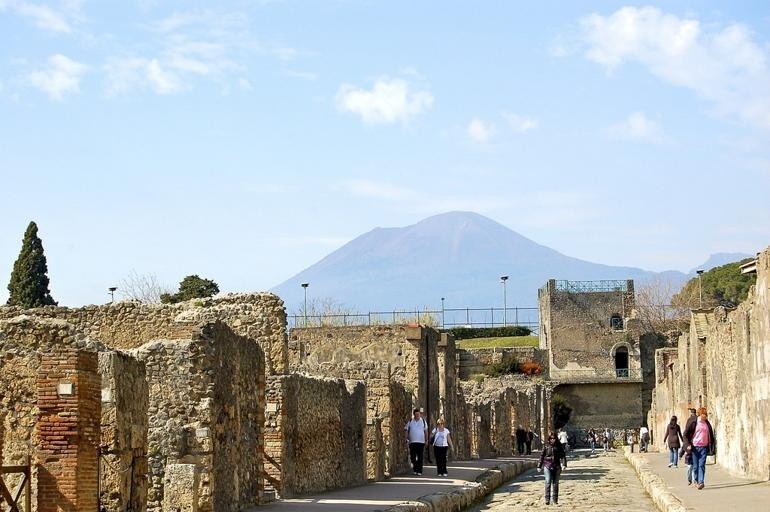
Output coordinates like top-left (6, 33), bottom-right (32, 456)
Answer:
top-left (430, 428), bottom-right (437, 445)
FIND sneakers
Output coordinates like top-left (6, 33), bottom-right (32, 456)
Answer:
top-left (417, 473), bottom-right (421, 476)
top-left (443, 474), bottom-right (448, 477)
top-left (413, 472), bottom-right (417, 475)
top-left (438, 474), bottom-right (441, 477)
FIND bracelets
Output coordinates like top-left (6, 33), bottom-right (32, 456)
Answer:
top-left (425, 440), bottom-right (428, 441)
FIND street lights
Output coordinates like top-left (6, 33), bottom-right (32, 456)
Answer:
top-left (500, 276), bottom-right (508, 328)
top-left (107, 287), bottom-right (116, 303)
top-left (696, 269), bottom-right (704, 309)
top-left (301, 283), bottom-right (309, 328)
top-left (440, 297), bottom-right (446, 331)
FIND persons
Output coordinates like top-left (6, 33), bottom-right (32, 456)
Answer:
top-left (525, 427), bottom-right (534, 455)
top-left (664, 407), bottom-right (715, 490)
top-left (404, 409), bottom-right (428, 476)
top-left (516, 424), bottom-right (527, 457)
top-left (587, 427), bottom-right (650, 453)
top-left (429, 418), bottom-right (453, 477)
top-left (537, 432), bottom-right (567, 506)
top-left (557, 428), bottom-right (569, 452)
